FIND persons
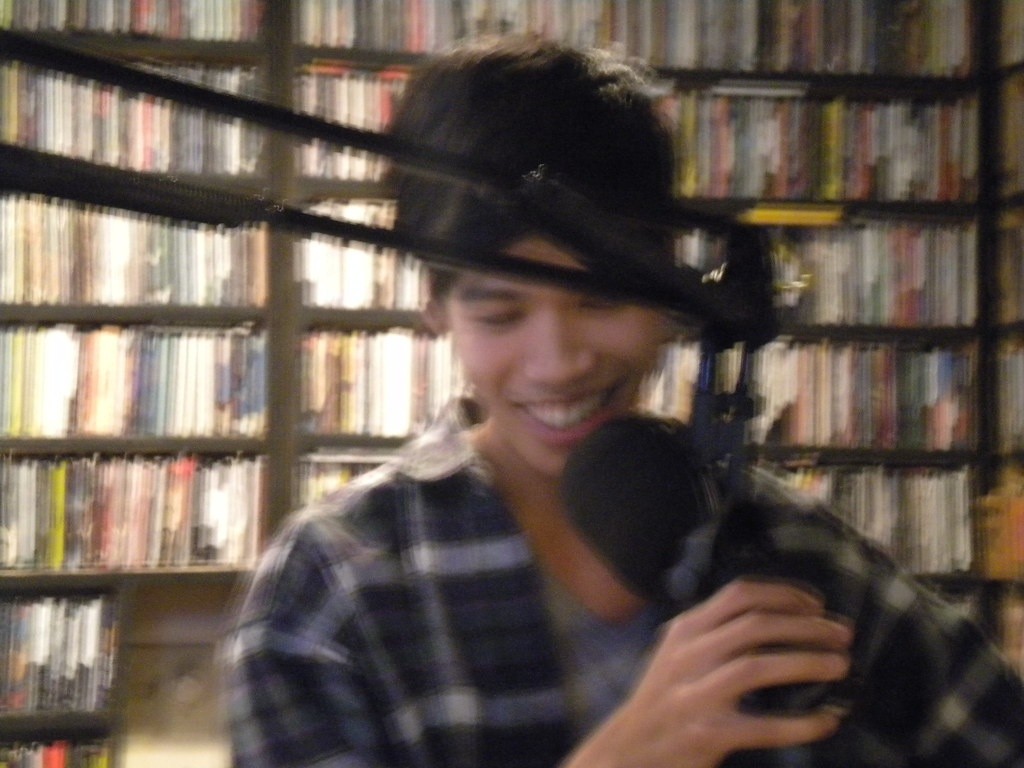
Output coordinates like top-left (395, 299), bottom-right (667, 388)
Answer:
top-left (216, 32), bottom-right (1024, 768)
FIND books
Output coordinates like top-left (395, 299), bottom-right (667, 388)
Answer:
top-left (289, 58), bottom-right (413, 183)
top-left (1, 191), bottom-right (269, 306)
top-left (977, 463), bottom-right (1023, 578)
top-left (1, 454), bottom-right (268, 572)
top-left (292, 444), bottom-right (389, 509)
top-left (649, 80), bottom-right (980, 201)
top-left (0, 50), bottom-right (265, 178)
top-left (296, 326), bottom-right (467, 438)
top-left (674, 204), bottom-right (982, 329)
top-left (998, 0), bottom-right (1024, 321)
top-left (996, 334), bottom-right (1024, 455)
top-left (787, 465), bottom-right (974, 578)
top-left (293, 194), bottom-right (435, 312)
top-left (992, 587), bottom-right (1024, 679)
top-left (0, 585), bottom-right (119, 768)
top-left (291, 0), bottom-right (978, 79)
top-left (634, 337), bottom-right (978, 456)
top-left (1, 0), bottom-right (264, 43)
top-left (2, 325), bottom-right (266, 441)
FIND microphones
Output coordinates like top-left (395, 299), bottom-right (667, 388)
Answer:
top-left (560, 413), bottom-right (828, 713)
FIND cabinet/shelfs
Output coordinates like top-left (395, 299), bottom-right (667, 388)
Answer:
top-left (0, 0), bottom-right (1024, 768)
